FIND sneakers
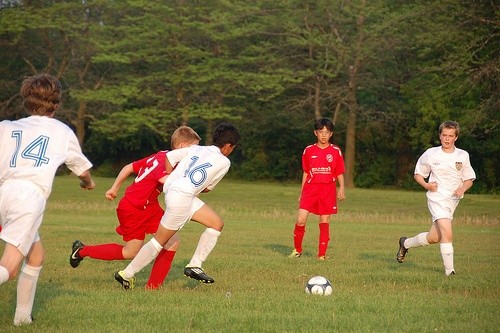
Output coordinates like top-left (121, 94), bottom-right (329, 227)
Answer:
top-left (70, 240), bottom-right (84, 268)
top-left (397, 237), bottom-right (409, 263)
top-left (288, 249), bottom-right (302, 258)
top-left (114, 270), bottom-right (136, 290)
top-left (449, 271), bottom-right (456, 277)
top-left (317, 256), bottom-right (326, 260)
top-left (184, 263), bottom-right (215, 285)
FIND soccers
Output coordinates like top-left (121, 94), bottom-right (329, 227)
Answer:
top-left (306, 276), bottom-right (333, 296)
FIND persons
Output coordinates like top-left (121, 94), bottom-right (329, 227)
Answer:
top-left (0, 72), bottom-right (95, 328)
top-left (69, 125), bottom-right (201, 291)
top-left (397, 120), bottom-right (476, 277)
top-left (114, 122), bottom-right (241, 291)
top-left (287, 119), bottom-right (345, 261)
